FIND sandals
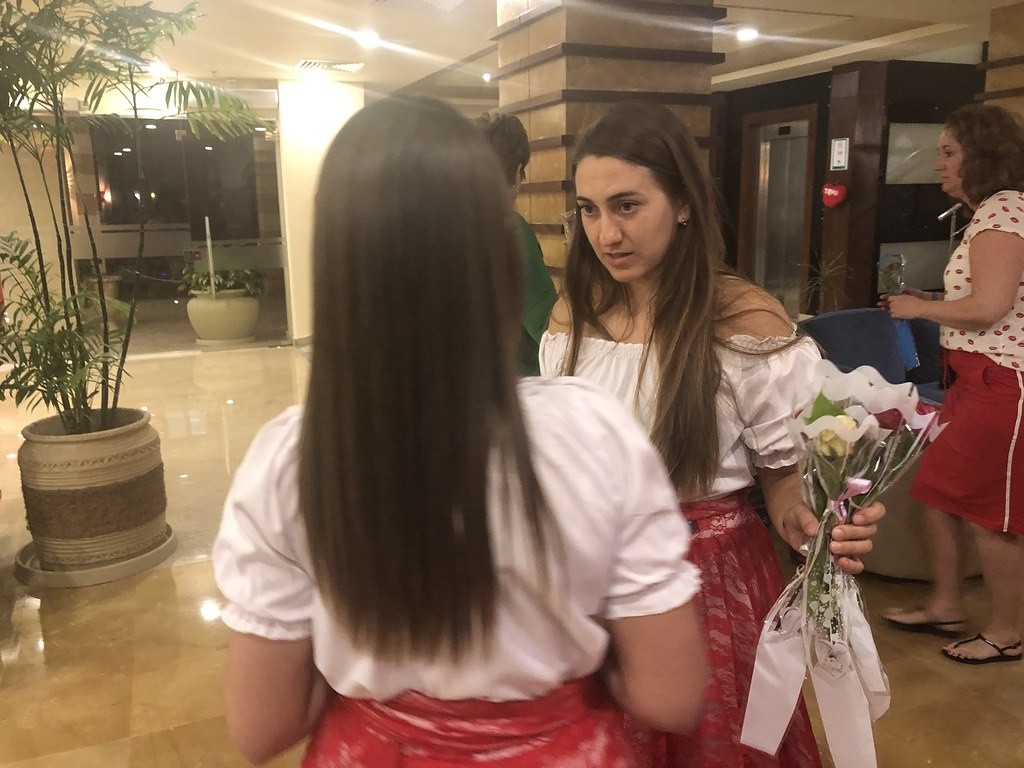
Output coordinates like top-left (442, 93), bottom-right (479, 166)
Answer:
top-left (942, 632), bottom-right (1022, 664)
top-left (883, 605), bottom-right (968, 638)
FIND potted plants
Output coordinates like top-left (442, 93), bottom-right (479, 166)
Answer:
top-left (179, 265), bottom-right (270, 348)
top-left (0, 0), bottom-right (282, 591)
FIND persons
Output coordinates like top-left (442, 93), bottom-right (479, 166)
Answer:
top-left (537, 101), bottom-right (885, 768)
top-left (465, 112), bottom-right (556, 381)
top-left (208, 96), bottom-right (710, 768)
top-left (875, 106), bottom-right (1024, 664)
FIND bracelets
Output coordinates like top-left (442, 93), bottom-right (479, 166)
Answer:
top-left (931, 291), bottom-right (939, 301)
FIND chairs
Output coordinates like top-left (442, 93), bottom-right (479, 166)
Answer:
top-left (795, 306), bottom-right (945, 403)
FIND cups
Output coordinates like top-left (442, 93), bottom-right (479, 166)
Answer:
top-left (875, 252), bottom-right (906, 295)
top-left (560, 208), bottom-right (577, 238)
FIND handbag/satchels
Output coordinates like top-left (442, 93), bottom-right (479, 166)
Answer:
top-left (896, 291), bottom-right (941, 384)
top-left (803, 574), bottom-right (876, 768)
top-left (834, 578), bottom-right (891, 723)
top-left (741, 574), bottom-right (811, 754)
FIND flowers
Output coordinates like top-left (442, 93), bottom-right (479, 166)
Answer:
top-left (767, 359), bottom-right (951, 675)
